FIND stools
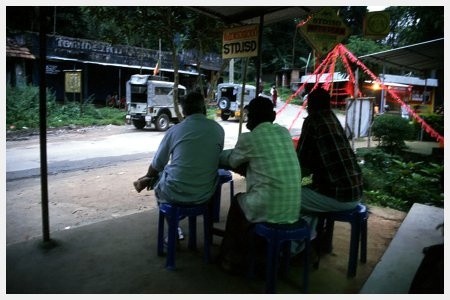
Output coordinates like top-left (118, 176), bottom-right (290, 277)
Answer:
top-left (313, 202), bottom-right (369, 277)
top-left (157, 201), bottom-right (214, 270)
top-left (214, 168), bottom-right (235, 222)
top-left (241, 217), bottom-right (311, 295)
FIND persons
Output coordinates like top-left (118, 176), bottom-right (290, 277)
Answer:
top-left (295, 87), bottom-right (365, 270)
top-left (133, 92), bottom-right (225, 246)
top-left (270, 85), bottom-right (275, 97)
top-left (106, 91), bottom-right (126, 109)
top-left (272, 86), bottom-right (278, 107)
top-left (206, 96), bottom-right (302, 272)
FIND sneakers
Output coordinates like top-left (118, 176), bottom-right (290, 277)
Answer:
top-left (133, 176), bottom-right (151, 193)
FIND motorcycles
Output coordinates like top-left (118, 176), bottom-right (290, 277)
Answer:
top-left (213, 83), bottom-right (256, 123)
top-left (125, 74), bottom-right (187, 133)
